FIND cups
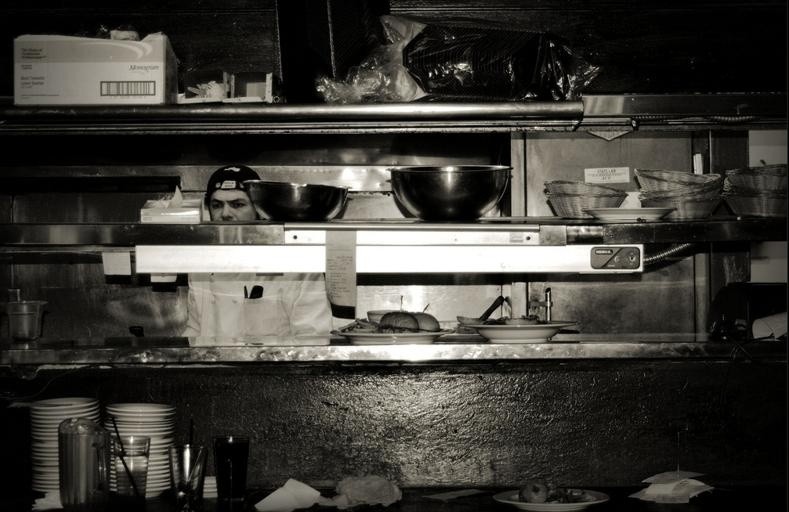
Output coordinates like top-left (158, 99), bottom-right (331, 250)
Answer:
top-left (165, 441), bottom-right (208, 512)
top-left (2, 300), bottom-right (49, 342)
top-left (210, 433), bottom-right (251, 504)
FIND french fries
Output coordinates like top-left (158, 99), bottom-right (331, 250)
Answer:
top-left (331, 318), bottom-right (380, 333)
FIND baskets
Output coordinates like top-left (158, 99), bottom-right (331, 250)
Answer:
top-left (542, 157), bottom-right (789, 219)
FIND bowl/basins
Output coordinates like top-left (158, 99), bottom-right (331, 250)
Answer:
top-left (386, 158), bottom-right (513, 222)
top-left (366, 307), bottom-right (404, 323)
top-left (236, 177), bottom-right (352, 220)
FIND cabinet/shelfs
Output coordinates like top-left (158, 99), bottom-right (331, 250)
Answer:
top-left (0, 92), bottom-right (788, 370)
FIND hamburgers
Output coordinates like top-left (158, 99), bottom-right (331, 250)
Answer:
top-left (380, 310), bottom-right (418, 334)
top-left (415, 313), bottom-right (439, 333)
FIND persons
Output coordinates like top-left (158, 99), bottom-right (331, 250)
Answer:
top-left (184, 165), bottom-right (333, 344)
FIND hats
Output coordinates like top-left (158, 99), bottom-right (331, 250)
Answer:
top-left (205, 166), bottom-right (261, 220)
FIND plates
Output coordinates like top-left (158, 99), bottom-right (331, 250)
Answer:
top-left (461, 317), bottom-right (579, 342)
top-left (328, 325), bottom-right (455, 343)
top-left (581, 206), bottom-right (678, 221)
top-left (24, 394), bottom-right (177, 501)
top-left (201, 475), bottom-right (217, 499)
top-left (490, 487), bottom-right (611, 512)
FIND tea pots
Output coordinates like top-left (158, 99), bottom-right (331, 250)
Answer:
top-left (56, 417), bottom-right (111, 512)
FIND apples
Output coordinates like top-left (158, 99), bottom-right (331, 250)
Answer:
top-left (520, 482), bottom-right (549, 503)
